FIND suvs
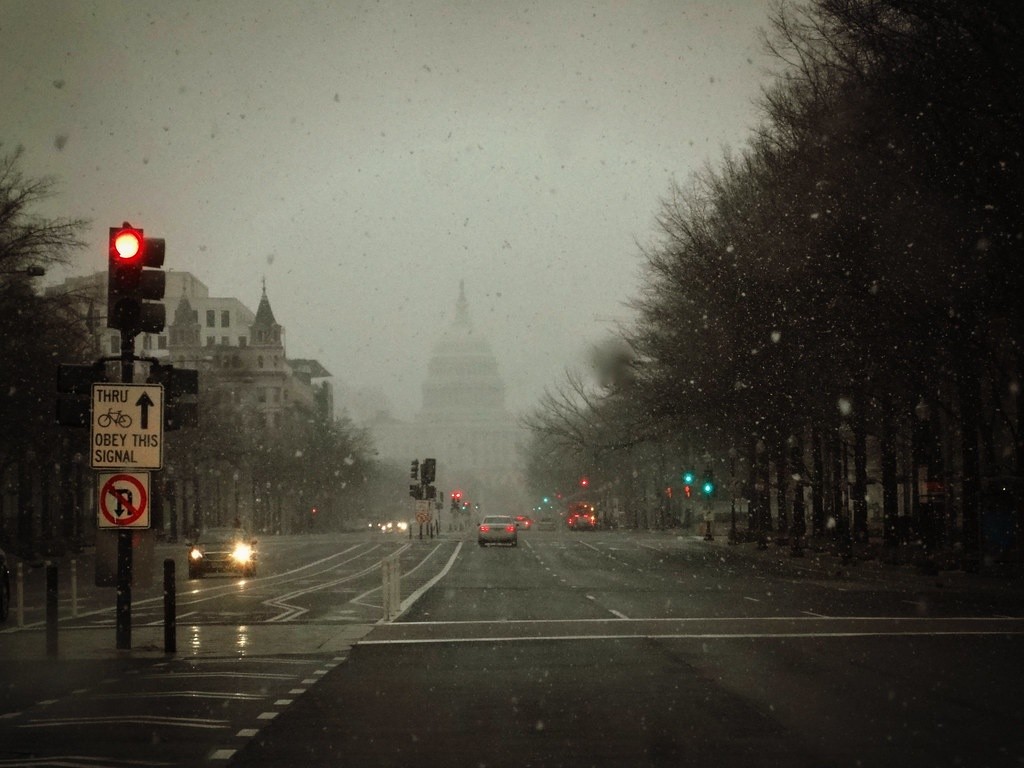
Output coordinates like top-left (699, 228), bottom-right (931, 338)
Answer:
top-left (567, 502), bottom-right (598, 531)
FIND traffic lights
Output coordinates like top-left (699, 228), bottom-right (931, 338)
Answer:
top-left (455, 493), bottom-right (461, 510)
top-left (106, 221), bottom-right (144, 336)
top-left (409, 459), bottom-right (419, 480)
top-left (434, 502), bottom-right (438, 509)
top-left (440, 504), bottom-right (444, 510)
top-left (464, 501), bottom-right (469, 508)
top-left (57, 359), bottom-right (114, 436)
top-left (427, 485), bottom-right (437, 498)
top-left (683, 472), bottom-right (695, 498)
top-left (452, 492), bottom-right (455, 511)
top-left (148, 365), bottom-right (200, 431)
top-left (703, 469), bottom-right (713, 500)
top-left (409, 482), bottom-right (419, 502)
top-left (580, 478), bottom-right (589, 486)
top-left (144, 234), bottom-right (168, 334)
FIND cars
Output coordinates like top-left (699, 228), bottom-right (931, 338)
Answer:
top-left (537, 517), bottom-right (557, 531)
top-left (514, 516), bottom-right (530, 530)
top-left (187, 527), bottom-right (260, 579)
top-left (476, 515), bottom-right (518, 547)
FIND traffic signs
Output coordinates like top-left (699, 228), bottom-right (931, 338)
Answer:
top-left (92, 382), bottom-right (163, 471)
